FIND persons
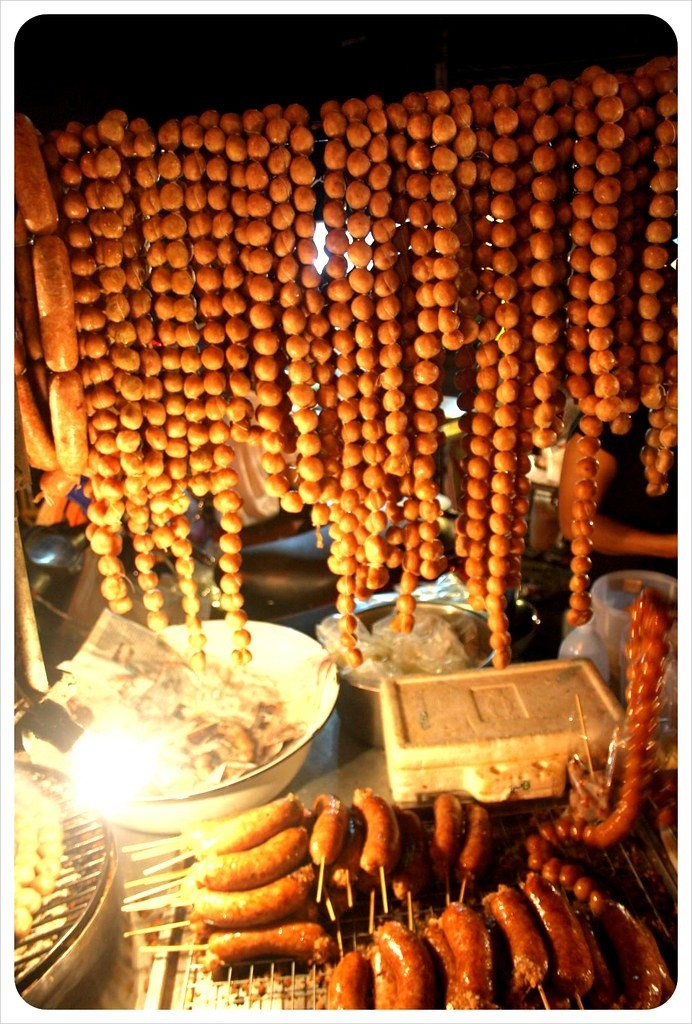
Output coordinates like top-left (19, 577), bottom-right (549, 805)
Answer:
top-left (203, 442), bottom-right (306, 546)
top-left (558, 396), bottom-right (679, 590)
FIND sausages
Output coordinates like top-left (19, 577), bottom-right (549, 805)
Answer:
top-left (185, 787), bottom-right (678, 1010)
top-left (14, 115), bottom-right (88, 507)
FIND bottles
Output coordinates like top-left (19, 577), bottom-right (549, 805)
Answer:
top-left (559, 572), bottom-right (678, 770)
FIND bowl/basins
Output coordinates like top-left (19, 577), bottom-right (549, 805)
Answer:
top-left (335, 602), bottom-right (496, 748)
top-left (20, 619), bottom-right (340, 834)
